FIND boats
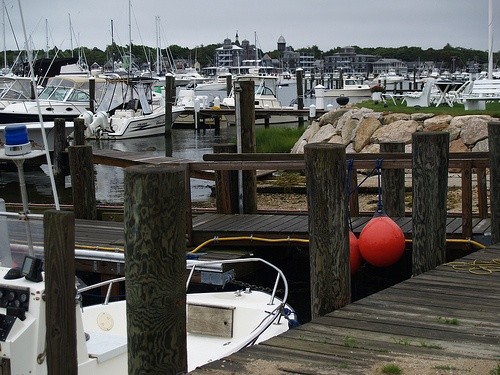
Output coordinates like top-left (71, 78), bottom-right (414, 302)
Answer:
top-left (1, 1), bottom-right (500, 120)
top-left (69, 1), bottom-right (185, 138)
top-left (0, 251), bottom-right (297, 375)
top-left (1, 77), bottom-right (98, 151)
